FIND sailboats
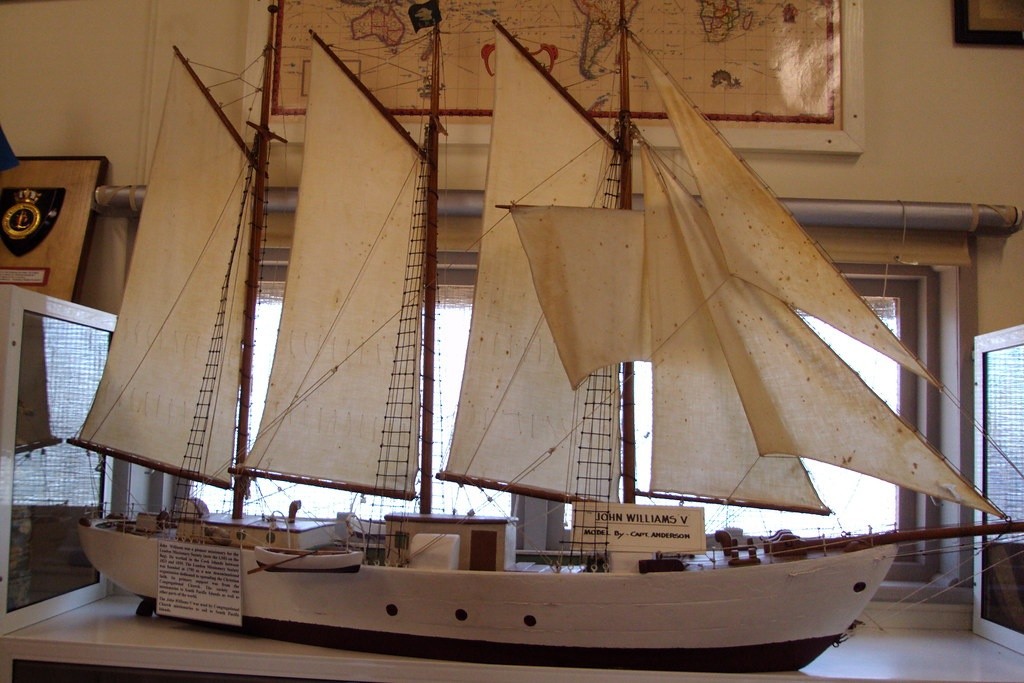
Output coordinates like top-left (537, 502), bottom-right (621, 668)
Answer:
top-left (66, 19), bottom-right (1024, 671)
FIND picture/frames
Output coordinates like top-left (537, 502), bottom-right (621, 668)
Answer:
top-left (951, 0), bottom-right (1024, 48)
top-left (0, 154), bottom-right (110, 302)
top-left (242, 0), bottom-right (866, 156)
